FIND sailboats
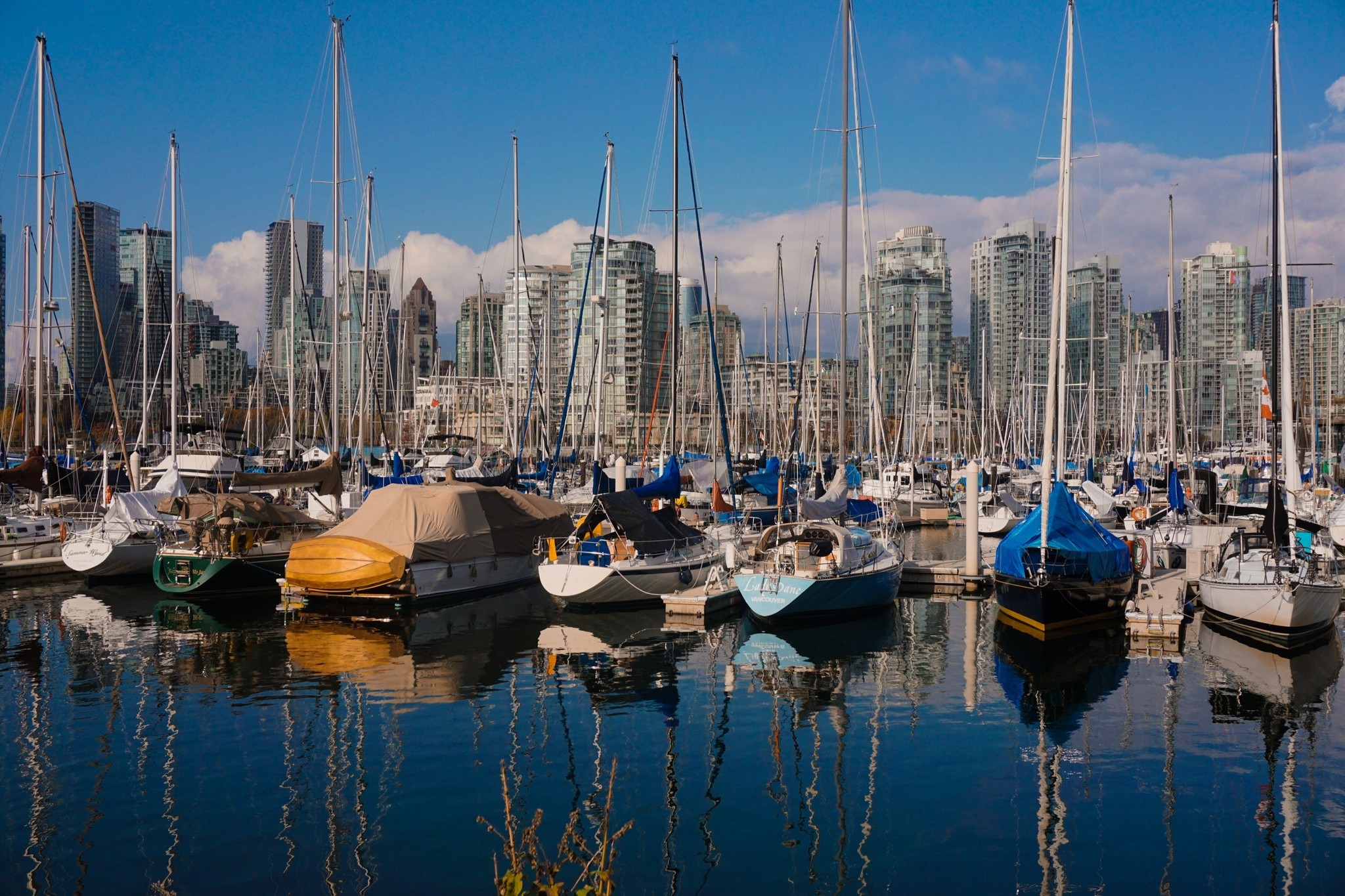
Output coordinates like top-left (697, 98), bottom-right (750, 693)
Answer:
top-left (0, 2), bottom-right (1345, 595)
top-left (723, 0), bottom-right (906, 622)
top-left (989, 0), bottom-right (1138, 633)
top-left (530, 38), bottom-right (743, 605)
top-left (1197, 1), bottom-right (1345, 653)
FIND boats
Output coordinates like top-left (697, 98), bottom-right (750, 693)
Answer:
top-left (277, 466), bottom-right (602, 617)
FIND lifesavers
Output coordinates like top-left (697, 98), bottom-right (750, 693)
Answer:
top-left (955, 484), bottom-right (964, 491)
top-left (1132, 506), bottom-right (1147, 521)
top-left (106, 484), bottom-right (112, 503)
top-left (1302, 490), bottom-right (1312, 501)
top-left (228, 635), bottom-right (253, 662)
top-left (60, 521), bottom-right (66, 542)
top-left (231, 527), bottom-right (254, 552)
top-left (675, 504), bottom-right (681, 519)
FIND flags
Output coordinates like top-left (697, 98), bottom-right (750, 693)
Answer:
top-left (1261, 368), bottom-right (1273, 420)
top-left (1229, 271), bottom-right (1237, 284)
top-left (431, 399), bottom-right (440, 407)
